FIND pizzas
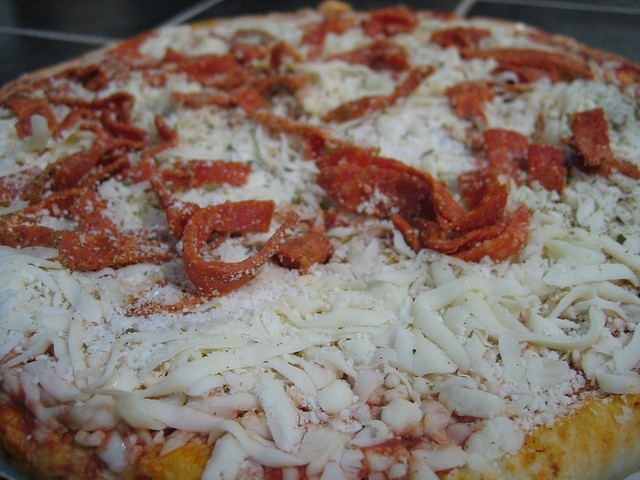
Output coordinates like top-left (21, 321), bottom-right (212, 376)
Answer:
top-left (1, 0), bottom-right (640, 480)
